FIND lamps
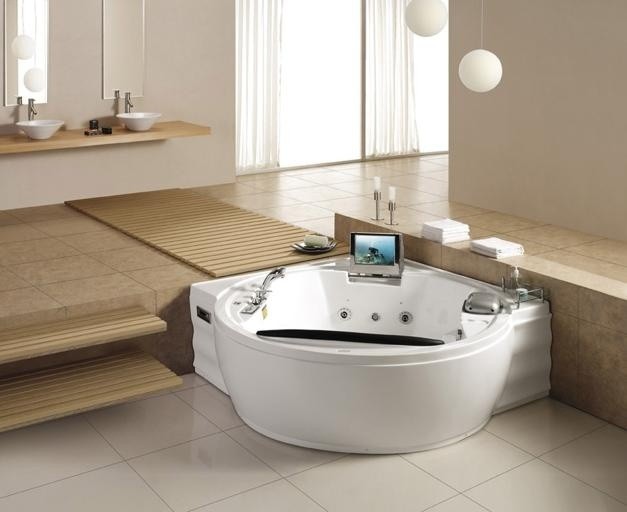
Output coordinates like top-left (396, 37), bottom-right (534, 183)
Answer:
top-left (6, 0), bottom-right (45, 97)
top-left (404, 0), bottom-right (504, 96)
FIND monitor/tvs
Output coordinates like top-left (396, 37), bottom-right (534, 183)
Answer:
top-left (348, 232), bottom-right (404, 277)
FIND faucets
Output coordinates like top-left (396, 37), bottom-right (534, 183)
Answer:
top-left (114, 90), bottom-right (119, 98)
top-left (28, 99), bottom-right (37, 120)
top-left (17, 97), bottom-right (22, 104)
top-left (263, 267), bottom-right (286, 291)
top-left (125, 92), bottom-right (133, 113)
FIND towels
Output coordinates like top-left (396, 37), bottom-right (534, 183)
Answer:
top-left (421, 217), bottom-right (526, 261)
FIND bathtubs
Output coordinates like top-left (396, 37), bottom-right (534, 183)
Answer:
top-left (188, 259), bottom-right (553, 456)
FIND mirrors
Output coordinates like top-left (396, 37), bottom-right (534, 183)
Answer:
top-left (3, 0), bottom-right (49, 110)
top-left (101, 0), bottom-right (146, 101)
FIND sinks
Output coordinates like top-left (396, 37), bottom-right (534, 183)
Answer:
top-left (16, 120), bottom-right (64, 139)
top-left (115, 113), bottom-right (162, 131)
